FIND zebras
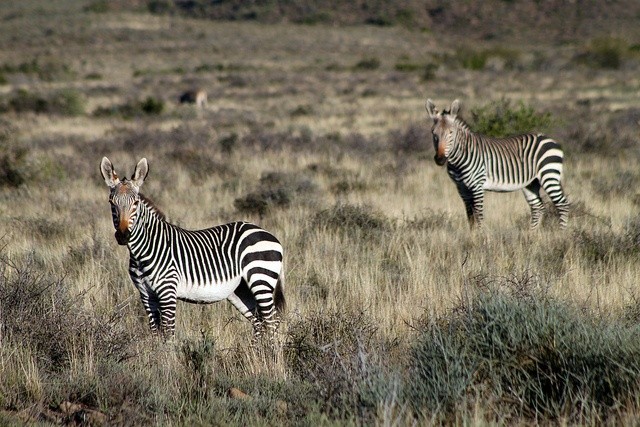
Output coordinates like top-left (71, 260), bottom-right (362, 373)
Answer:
top-left (100, 156), bottom-right (288, 350)
top-left (426, 98), bottom-right (570, 237)
